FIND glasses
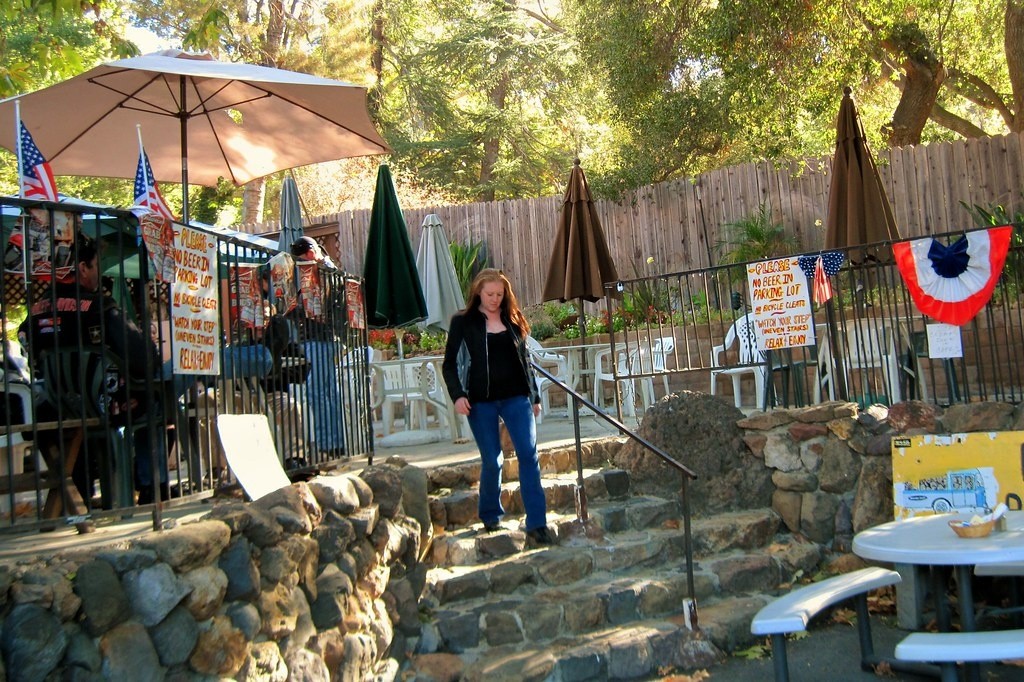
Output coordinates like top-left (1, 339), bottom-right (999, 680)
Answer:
top-left (81, 232), bottom-right (90, 248)
top-left (290, 242), bottom-right (309, 250)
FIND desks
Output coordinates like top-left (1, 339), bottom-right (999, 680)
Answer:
top-left (534, 343), bottom-right (626, 416)
top-left (814, 316), bottom-right (927, 408)
top-left (852, 514), bottom-right (1024, 682)
top-left (344, 356), bottom-right (445, 447)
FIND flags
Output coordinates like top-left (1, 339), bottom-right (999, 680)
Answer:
top-left (127, 147), bottom-right (179, 223)
top-left (6, 117), bottom-right (123, 209)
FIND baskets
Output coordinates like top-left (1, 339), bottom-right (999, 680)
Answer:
top-left (948, 519), bottom-right (995, 538)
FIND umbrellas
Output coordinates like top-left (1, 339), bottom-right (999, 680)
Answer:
top-left (416, 209), bottom-right (467, 337)
top-left (543, 157), bottom-right (624, 395)
top-left (278, 171), bottom-right (305, 251)
top-left (361, 160), bottom-right (431, 359)
top-left (0, 47), bottom-right (398, 226)
top-left (824, 85), bottom-right (903, 319)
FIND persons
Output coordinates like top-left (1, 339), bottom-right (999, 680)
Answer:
top-left (288, 236), bottom-right (347, 458)
top-left (499, 348), bottom-right (541, 459)
top-left (180, 381), bottom-right (217, 462)
top-left (443, 268), bottom-right (561, 546)
top-left (150, 323), bottom-right (158, 342)
top-left (17, 232), bottom-right (290, 511)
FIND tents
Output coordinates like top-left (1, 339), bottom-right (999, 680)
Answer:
top-left (1, 193), bottom-right (280, 278)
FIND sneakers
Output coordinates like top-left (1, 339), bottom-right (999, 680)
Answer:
top-left (527, 526), bottom-right (551, 544)
top-left (484, 522), bottom-right (498, 531)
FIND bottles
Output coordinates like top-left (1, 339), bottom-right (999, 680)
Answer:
top-left (985, 508), bottom-right (1006, 532)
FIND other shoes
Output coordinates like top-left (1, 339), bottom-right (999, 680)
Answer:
top-left (263, 314), bottom-right (289, 376)
top-left (138, 480), bottom-right (178, 505)
top-left (286, 458), bottom-right (312, 480)
top-left (329, 448), bottom-right (346, 457)
top-left (204, 467), bottom-right (224, 478)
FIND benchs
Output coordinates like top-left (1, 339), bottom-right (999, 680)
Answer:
top-left (973, 564), bottom-right (1024, 628)
top-left (0, 417), bottom-right (112, 534)
top-left (894, 628), bottom-right (1024, 682)
top-left (749, 566), bottom-right (902, 682)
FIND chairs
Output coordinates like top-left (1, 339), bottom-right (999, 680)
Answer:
top-left (710, 311), bottom-right (901, 407)
top-left (335, 336), bottom-right (677, 447)
